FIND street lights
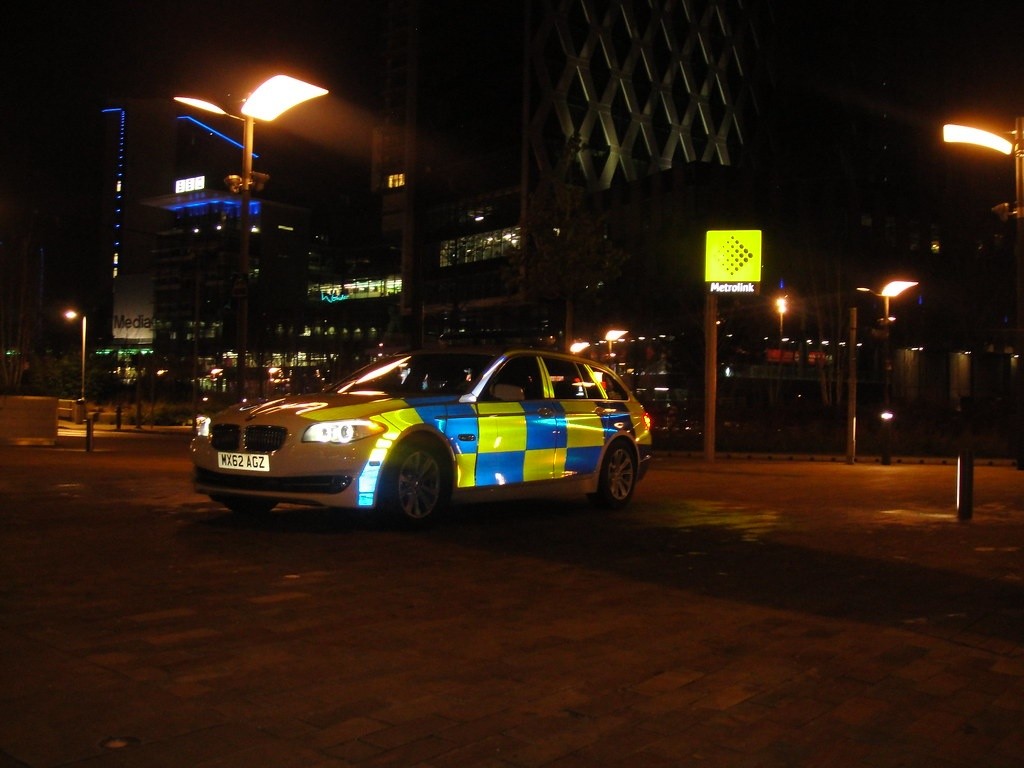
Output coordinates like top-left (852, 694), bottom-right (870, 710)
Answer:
top-left (856, 279), bottom-right (920, 466)
top-left (941, 114), bottom-right (1023, 263)
top-left (66, 309), bottom-right (92, 404)
top-left (170, 73), bottom-right (330, 407)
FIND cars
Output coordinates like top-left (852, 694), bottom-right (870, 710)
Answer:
top-left (187, 344), bottom-right (655, 528)
